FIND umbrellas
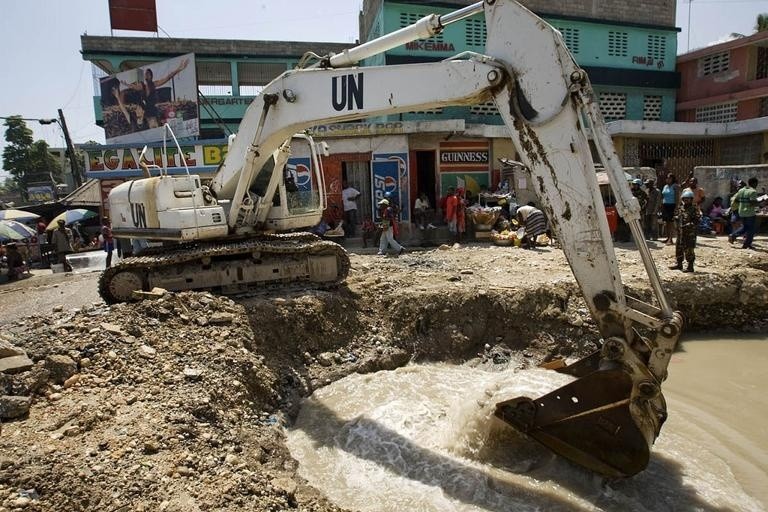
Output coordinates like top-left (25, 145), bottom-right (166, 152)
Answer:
top-left (46, 208), bottom-right (98, 232)
top-left (0, 219), bottom-right (37, 243)
top-left (0, 208), bottom-right (40, 221)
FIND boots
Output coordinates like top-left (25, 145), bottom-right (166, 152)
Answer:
top-left (668, 260), bottom-right (683, 270)
top-left (681, 260), bottom-right (695, 272)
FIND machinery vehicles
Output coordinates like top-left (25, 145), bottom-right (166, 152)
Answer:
top-left (98, 0), bottom-right (686, 483)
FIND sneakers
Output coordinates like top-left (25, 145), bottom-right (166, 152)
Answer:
top-left (418, 223), bottom-right (436, 231)
top-left (398, 246), bottom-right (404, 255)
top-left (743, 245), bottom-right (755, 249)
top-left (378, 252), bottom-right (386, 255)
top-left (728, 234), bottom-right (734, 244)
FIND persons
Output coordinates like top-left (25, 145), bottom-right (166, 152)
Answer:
top-left (6, 221), bottom-right (98, 281)
top-left (360, 211), bottom-right (383, 248)
top-left (99, 215), bottom-right (114, 268)
top-left (307, 214), bottom-right (331, 240)
top-left (52, 219), bottom-right (75, 273)
top-left (340, 177), bottom-right (361, 239)
top-left (667, 186), bottom-right (700, 273)
top-left (614, 168), bottom-right (766, 251)
top-left (375, 187), bottom-right (469, 255)
top-left (102, 56), bottom-right (190, 139)
top-left (514, 203), bottom-right (545, 250)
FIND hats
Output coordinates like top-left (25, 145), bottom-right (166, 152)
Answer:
top-left (643, 178), bottom-right (655, 185)
top-left (377, 199), bottom-right (391, 206)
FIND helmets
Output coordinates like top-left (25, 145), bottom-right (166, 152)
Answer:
top-left (632, 178), bottom-right (643, 186)
top-left (681, 191), bottom-right (695, 201)
